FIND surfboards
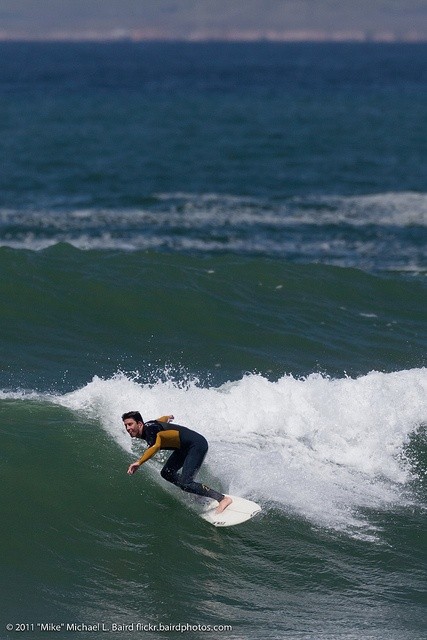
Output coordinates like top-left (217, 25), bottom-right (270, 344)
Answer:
top-left (198, 492), bottom-right (262, 528)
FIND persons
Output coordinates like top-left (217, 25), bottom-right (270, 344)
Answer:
top-left (121, 411), bottom-right (232, 515)
